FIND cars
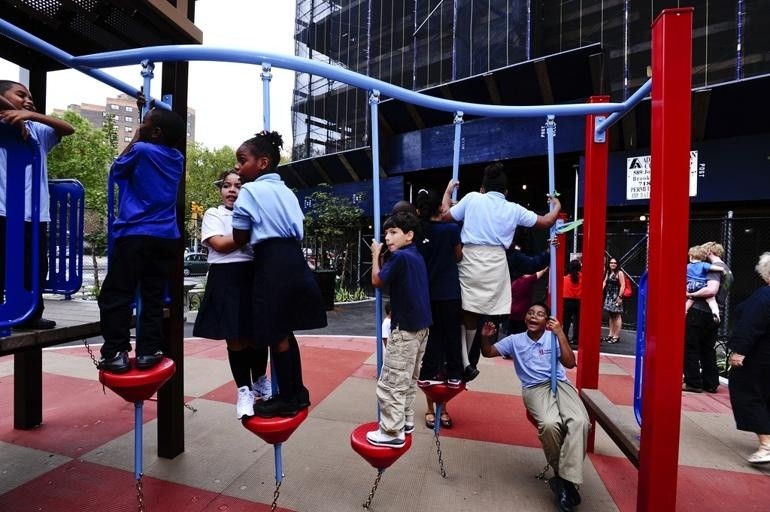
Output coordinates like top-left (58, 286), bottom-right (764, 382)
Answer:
top-left (184, 253), bottom-right (208, 277)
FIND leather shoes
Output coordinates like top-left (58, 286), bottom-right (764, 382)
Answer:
top-left (98, 352), bottom-right (131, 373)
top-left (424, 412), bottom-right (453, 430)
top-left (136, 354), bottom-right (165, 371)
top-left (549, 475), bottom-right (581, 512)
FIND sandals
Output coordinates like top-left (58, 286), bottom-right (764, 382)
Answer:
top-left (602, 335), bottom-right (620, 344)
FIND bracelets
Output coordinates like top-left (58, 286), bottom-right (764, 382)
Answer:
top-left (618, 295), bottom-right (623, 300)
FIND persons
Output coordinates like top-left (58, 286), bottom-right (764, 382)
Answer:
top-left (685, 245), bottom-right (727, 325)
top-left (681, 242), bottom-right (725, 393)
top-left (380, 163), bottom-right (562, 428)
top-left (193, 170), bottom-right (280, 420)
top-left (0, 79), bottom-right (75, 330)
top-left (561, 260), bottom-right (583, 350)
top-left (726, 251), bottom-right (770, 463)
top-left (365, 211), bottom-right (433, 449)
top-left (603, 257), bottom-right (626, 344)
top-left (96, 85), bottom-right (187, 374)
top-left (230, 130), bottom-right (328, 419)
top-left (480, 301), bottom-right (590, 511)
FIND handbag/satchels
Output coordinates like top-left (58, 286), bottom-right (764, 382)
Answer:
top-left (620, 276), bottom-right (633, 297)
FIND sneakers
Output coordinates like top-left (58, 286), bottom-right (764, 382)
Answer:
top-left (682, 379), bottom-right (719, 393)
top-left (36, 317), bottom-right (56, 331)
top-left (748, 443), bottom-right (770, 463)
top-left (236, 376), bottom-right (311, 420)
top-left (366, 421), bottom-right (415, 448)
top-left (417, 365), bottom-right (479, 389)
